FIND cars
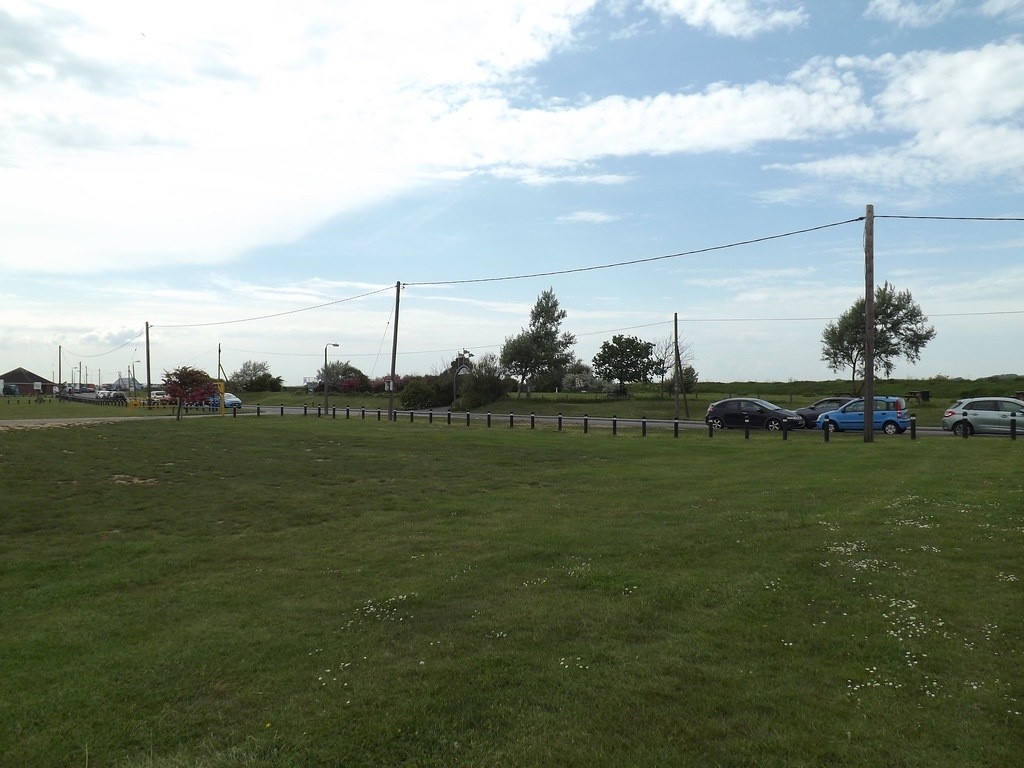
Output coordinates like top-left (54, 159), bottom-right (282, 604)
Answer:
top-left (793, 397), bottom-right (879, 430)
top-left (64, 385), bottom-right (94, 394)
top-left (817, 396), bottom-right (910, 435)
top-left (706, 398), bottom-right (805, 431)
top-left (211, 393), bottom-right (242, 409)
top-left (106, 387), bottom-right (111, 391)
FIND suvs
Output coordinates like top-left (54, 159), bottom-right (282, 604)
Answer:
top-left (151, 391), bottom-right (171, 399)
top-left (96, 391), bottom-right (128, 402)
top-left (942, 397), bottom-right (1024, 435)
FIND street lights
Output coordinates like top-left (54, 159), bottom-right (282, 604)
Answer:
top-left (324, 343), bottom-right (339, 414)
top-left (132, 361), bottom-right (141, 399)
